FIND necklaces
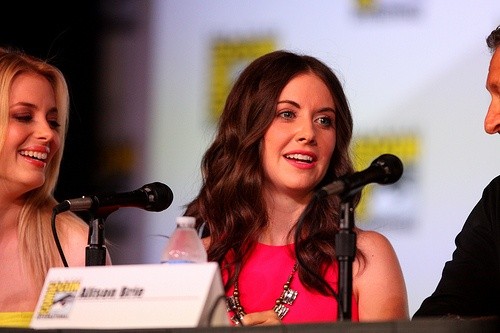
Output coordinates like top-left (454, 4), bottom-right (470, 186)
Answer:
top-left (226, 250), bottom-right (299, 326)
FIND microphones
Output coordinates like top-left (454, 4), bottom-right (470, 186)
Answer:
top-left (52, 182), bottom-right (174, 215)
top-left (313, 154), bottom-right (404, 202)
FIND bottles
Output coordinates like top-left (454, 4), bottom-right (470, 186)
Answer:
top-left (160, 216), bottom-right (207, 264)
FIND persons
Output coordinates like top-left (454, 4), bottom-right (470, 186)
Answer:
top-left (177, 51), bottom-right (410, 328)
top-left (0, 51), bottom-right (113, 328)
top-left (412, 25), bottom-right (500, 317)
